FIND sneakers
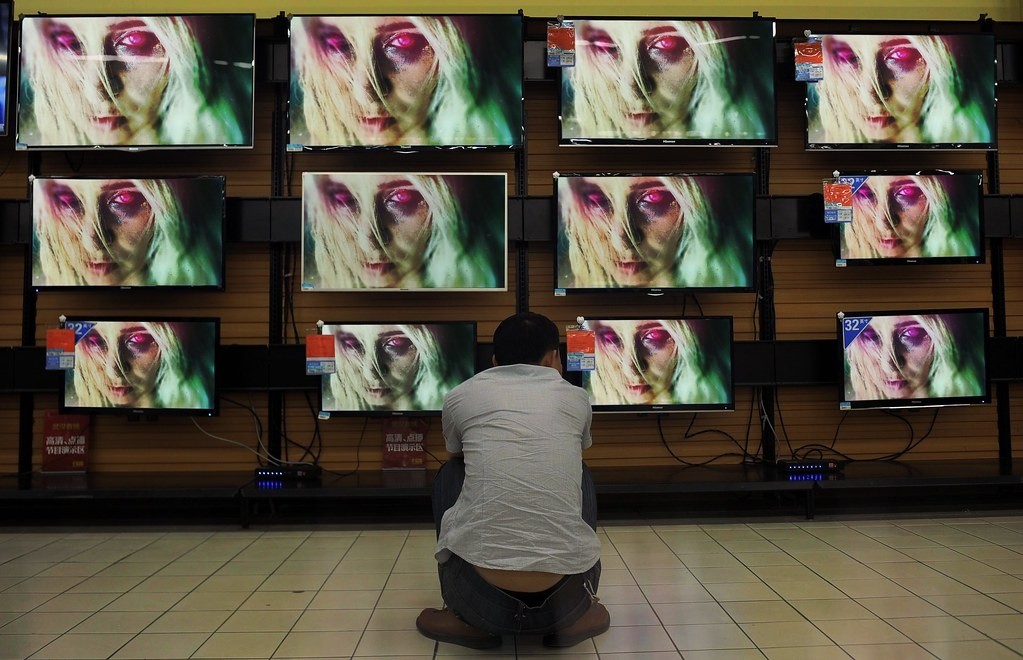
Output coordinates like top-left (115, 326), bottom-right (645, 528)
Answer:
top-left (416, 608), bottom-right (502, 648)
top-left (543, 602), bottom-right (611, 646)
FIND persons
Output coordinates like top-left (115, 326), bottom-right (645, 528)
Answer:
top-left (804, 35), bottom-right (991, 143)
top-left (843, 311), bottom-right (986, 401)
top-left (416, 312), bottom-right (610, 649)
top-left (291, 15), bottom-right (515, 146)
top-left (304, 173), bottom-right (505, 289)
top-left (65, 320), bottom-right (210, 409)
top-left (840, 173), bottom-right (976, 259)
top-left (557, 175), bottom-right (748, 288)
top-left (581, 319), bottom-right (728, 405)
top-left (33, 179), bottom-right (219, 286)
top-left (18, 15), bottom-right (243, 143)
top-left (321, 324), bottom-right (457, 411)
top-left (560, 21), bottom-right (768, 139)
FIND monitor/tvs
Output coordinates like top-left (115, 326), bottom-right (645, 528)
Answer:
top-left (0, 0), bottom-right (1001, 418)
